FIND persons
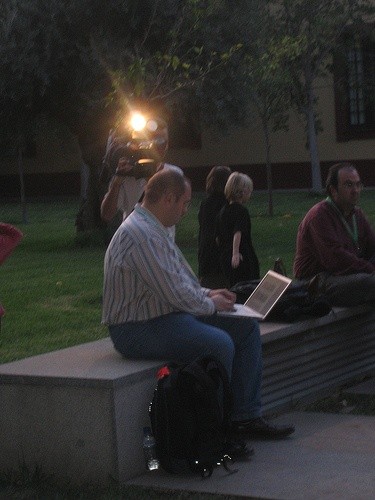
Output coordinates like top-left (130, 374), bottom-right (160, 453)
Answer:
top-left (215, 171), bottom-right (260, 289)
top-left (198, 166), bottom-right (232, 290)
top-left (100, 116), bottom-right (183, 241)
top-left (101, 170), bottom-right (296, 460)
top-left (290, 163), bottom-right (375, 308)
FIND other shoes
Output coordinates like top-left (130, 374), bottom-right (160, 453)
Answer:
top-left (234, 419), bottom-right (295, 437)
top-left (208, 431), bottom-right (254, 459)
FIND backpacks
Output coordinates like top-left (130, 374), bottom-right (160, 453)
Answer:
top-left (231, 266), bottom-right (336, 321)
top-left (149, 356), bottom-right (226, 472)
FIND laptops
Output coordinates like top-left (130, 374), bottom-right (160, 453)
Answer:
top-left (216, 269), bottom-right (292, 320)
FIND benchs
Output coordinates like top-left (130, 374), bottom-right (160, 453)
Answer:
top-left (0, 300), bottom-right (375, 493)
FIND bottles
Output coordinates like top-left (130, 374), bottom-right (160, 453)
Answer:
top-left (142, 427), bottom-right (160, 471)
